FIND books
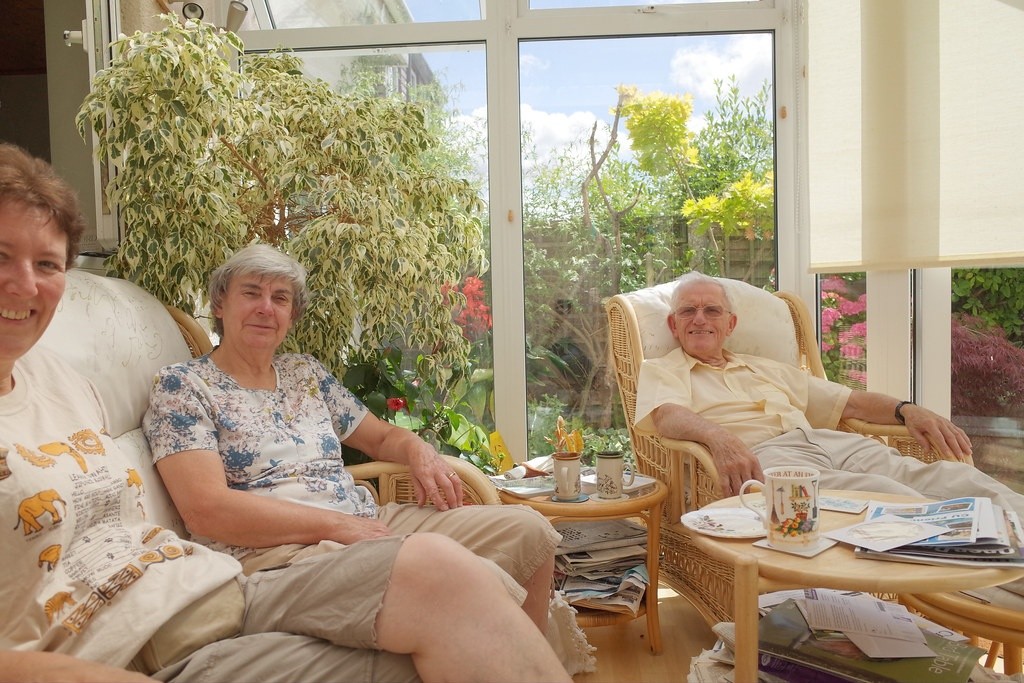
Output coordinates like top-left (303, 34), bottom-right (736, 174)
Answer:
top-left (751, 595), bottom-right (987, 683)
top-left (853, 497), bottom-right (1024, 569)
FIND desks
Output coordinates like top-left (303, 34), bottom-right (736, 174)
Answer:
top-left (497, 465), bottom-right (668, 656)
top-left (685, 487), bottom-right (1024, 682)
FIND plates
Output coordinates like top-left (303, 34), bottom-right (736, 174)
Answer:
top-left (680, 508), bottom-right (767, 538)
top-left (582, 472), bottom-right (656, 492)
top-left (491, 474), bottom-right (554, 498)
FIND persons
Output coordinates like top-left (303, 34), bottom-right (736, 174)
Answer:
top-left (143, 245), bottom-right (563, 637)
top-left (0, 143), bottom-right (579, 683)
top-left (630, 275), bottom-right (1023, 507)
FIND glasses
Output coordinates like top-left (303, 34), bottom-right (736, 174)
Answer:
top-left (675, 305), bottom-right (728, 320)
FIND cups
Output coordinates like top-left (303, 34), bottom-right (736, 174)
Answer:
top-left (739, 466), bottom-right (821, 551)
top-left (551, 452), bottom-right (582, 500)
top-left (595, 450), bottom-right (634, 499)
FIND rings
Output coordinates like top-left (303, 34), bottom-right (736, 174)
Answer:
top-left (449, 472), bottom-right (455, 478)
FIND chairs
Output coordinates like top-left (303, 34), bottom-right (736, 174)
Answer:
top-left (601, 274), bottom-right (975, 614)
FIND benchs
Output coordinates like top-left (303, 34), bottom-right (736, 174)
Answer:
top-left (25, 261), bottom-right (506, 550)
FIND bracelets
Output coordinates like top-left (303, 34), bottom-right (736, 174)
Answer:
top-left (894, 401), bottom-right (917, 424)
